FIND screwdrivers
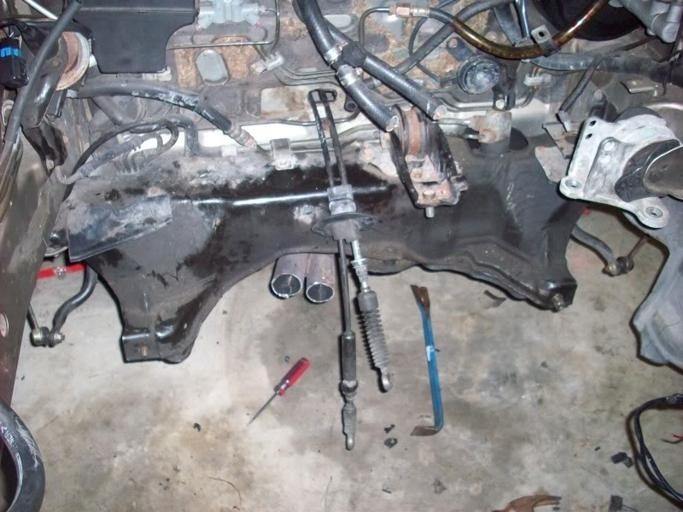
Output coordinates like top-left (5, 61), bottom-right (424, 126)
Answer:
top-left (246, 357), bottom-right (310, 425)
